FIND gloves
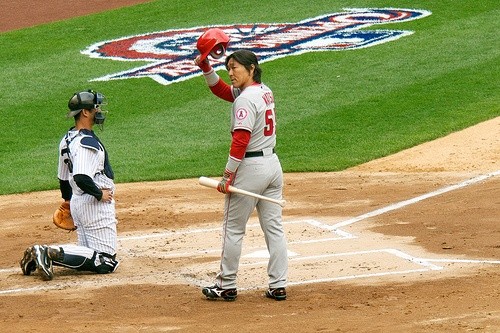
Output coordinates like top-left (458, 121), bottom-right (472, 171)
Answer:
top-left (194, 54), bottom-right (212, 73)
top-left (216, 168), bottom-right (234, 194)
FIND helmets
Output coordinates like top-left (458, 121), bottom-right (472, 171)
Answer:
top-left (197, 28), bottom-right (230, 64)
top-left (67, 92), bottom-right (94, 118)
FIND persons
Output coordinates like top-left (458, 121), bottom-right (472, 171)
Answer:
top-left (19, 90), bottom-right (119, 281)
top-left (195, 50), bottom-right (287, 301)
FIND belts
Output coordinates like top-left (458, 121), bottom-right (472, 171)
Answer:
top-left (245, 148), bottom-right (275, 157)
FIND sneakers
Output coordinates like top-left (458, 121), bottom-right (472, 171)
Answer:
top-left (19, 244), bottom-right (54, 280)
top-left (266, 287), bottom-right (287, 300)
top-left (202, 283), bottom-right (237, 301)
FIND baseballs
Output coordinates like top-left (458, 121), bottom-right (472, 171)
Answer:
top-left (199, 176), bottom-right (287, 208)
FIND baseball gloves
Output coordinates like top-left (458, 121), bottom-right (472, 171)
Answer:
top-left (52, 202), bottom-right (77, 233)
